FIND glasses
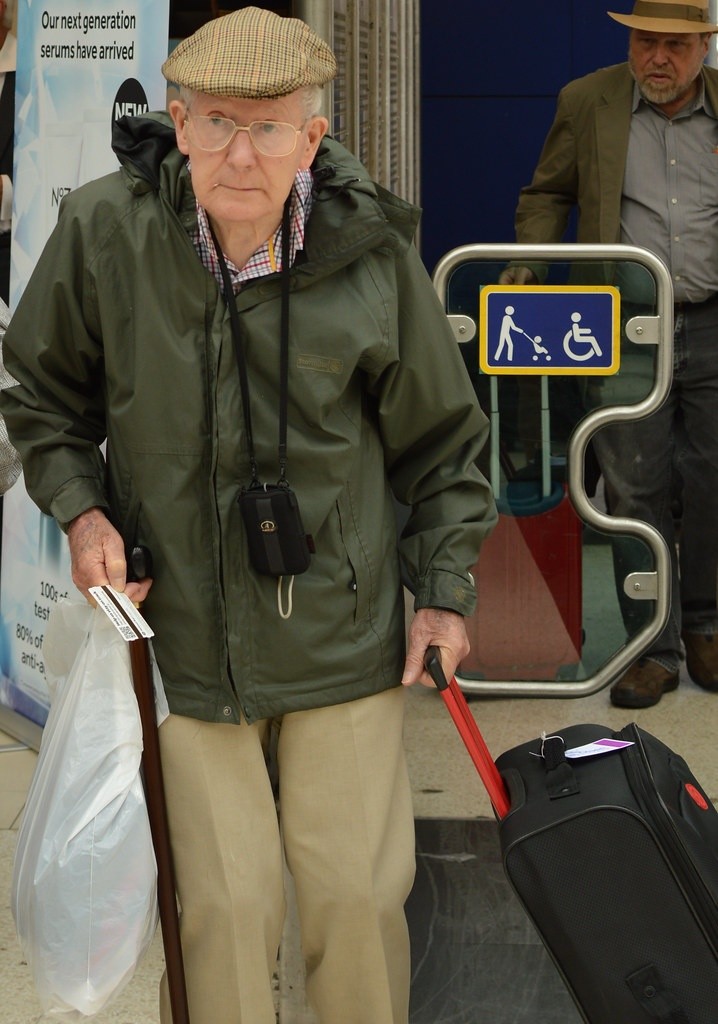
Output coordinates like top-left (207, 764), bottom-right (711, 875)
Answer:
top-left (183, 116), bottom-right (307, 157)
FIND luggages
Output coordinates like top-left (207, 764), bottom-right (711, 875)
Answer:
top-left (424, 646), bottom-right (718, 1024)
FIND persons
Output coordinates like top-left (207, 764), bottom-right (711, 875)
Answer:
top-left (1, 7), bottom-right (496, 1024)
top-left (498, 0), bottom-right (718, 711)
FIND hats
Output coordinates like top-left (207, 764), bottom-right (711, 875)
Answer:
top-left (606, 0), bottom-right (718, 33)
top-left (162, 6), bottom-right (336, 100)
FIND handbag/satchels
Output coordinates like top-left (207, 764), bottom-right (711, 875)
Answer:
top-left (10, 597), bottom-right (169, 1016)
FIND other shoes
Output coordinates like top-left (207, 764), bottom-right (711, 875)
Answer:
top-left (610, 655), bottom-right (679, 709)
top-left (685, 633), bottom-right (717, 693)
